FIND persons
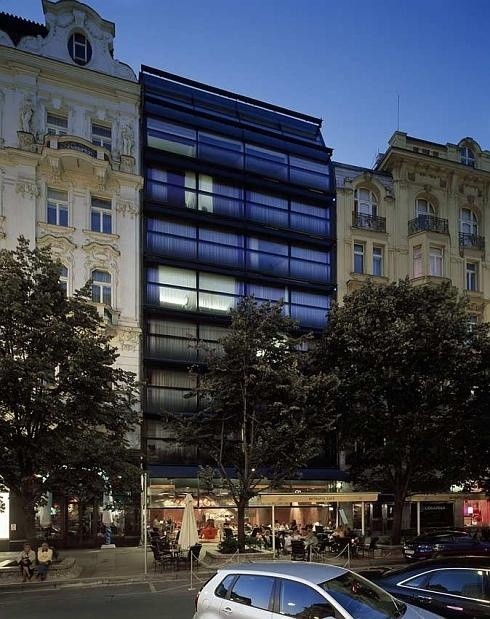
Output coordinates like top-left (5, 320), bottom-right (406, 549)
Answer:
top-left (15, 543), bottom-right (36, 583)
top-left (152, 515), bottom-right (214, 543)
top-left (223, 519), bottom-right (365, 559)
top-left (33, 542), bottom-right (54, 581)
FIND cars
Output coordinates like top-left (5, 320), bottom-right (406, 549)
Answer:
top-left (192, 561), bottom-right (446, 619)
top-left (366, 529), bottom-right (490, 619)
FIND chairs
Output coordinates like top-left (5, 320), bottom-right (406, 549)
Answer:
top-left (224, 520), bottom-right (381, 561)
top-left (149, 530), bottom-right (202, 572)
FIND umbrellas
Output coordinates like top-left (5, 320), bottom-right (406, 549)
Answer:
top-left (178, 493), bottom-right (201, 588)
